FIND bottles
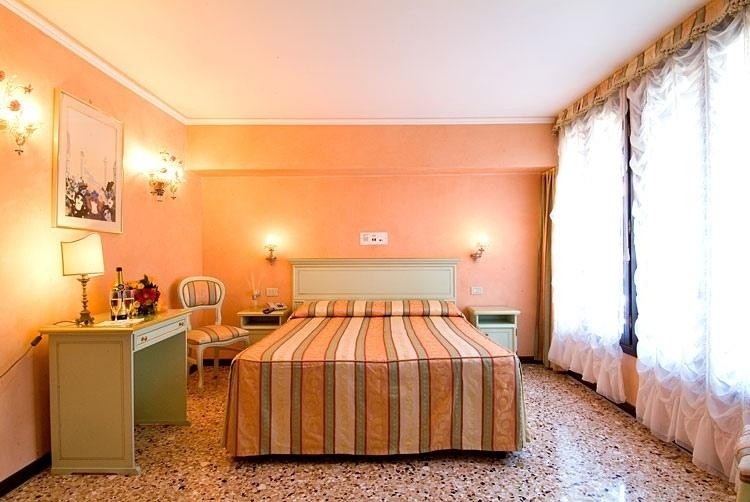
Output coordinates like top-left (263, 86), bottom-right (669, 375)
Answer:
top-left (114, 266), bottom-right (127, 320)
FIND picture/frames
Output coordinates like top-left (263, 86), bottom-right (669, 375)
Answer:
top-left (48, 86), bottom-right (124, 237)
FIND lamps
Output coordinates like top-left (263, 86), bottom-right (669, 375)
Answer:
top-left (148, 148), bottom-right (186, 203)
top-left (463, 228), bottom-right (493, 261)
top-left (262, 232), bottom-right (280, 265)
top-left (247, 285), bottom-right (262, 312)
top-left (1, 71), bottom-right (48, 155)
top-left (60, 230), bottom-right (108, 327)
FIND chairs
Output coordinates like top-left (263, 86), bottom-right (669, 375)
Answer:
top-left (177, 273), bottom-right (251, 391)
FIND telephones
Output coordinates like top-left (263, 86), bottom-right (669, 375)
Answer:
top-left (264, 302), bottom-right (288, 312)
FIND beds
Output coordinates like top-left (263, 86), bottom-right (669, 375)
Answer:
top-left (220, 256), bottom-right (531, 463)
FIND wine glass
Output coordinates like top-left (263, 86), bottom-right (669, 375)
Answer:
top-left (110, 288), bottom-right (122, 322)
top-left (122, 289), bottom-right (134, 323)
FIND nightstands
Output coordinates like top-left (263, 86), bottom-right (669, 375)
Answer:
top-left (234, 305), bottom-right (288, 345)
top-left (465, 304), bottom-right (522, 357)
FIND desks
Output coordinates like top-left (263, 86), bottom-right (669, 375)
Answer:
top-left (38, 306), bottom-right (191, 476)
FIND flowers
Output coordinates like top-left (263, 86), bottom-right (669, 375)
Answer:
top-left (112, 272), bottom-right (160, 315)
